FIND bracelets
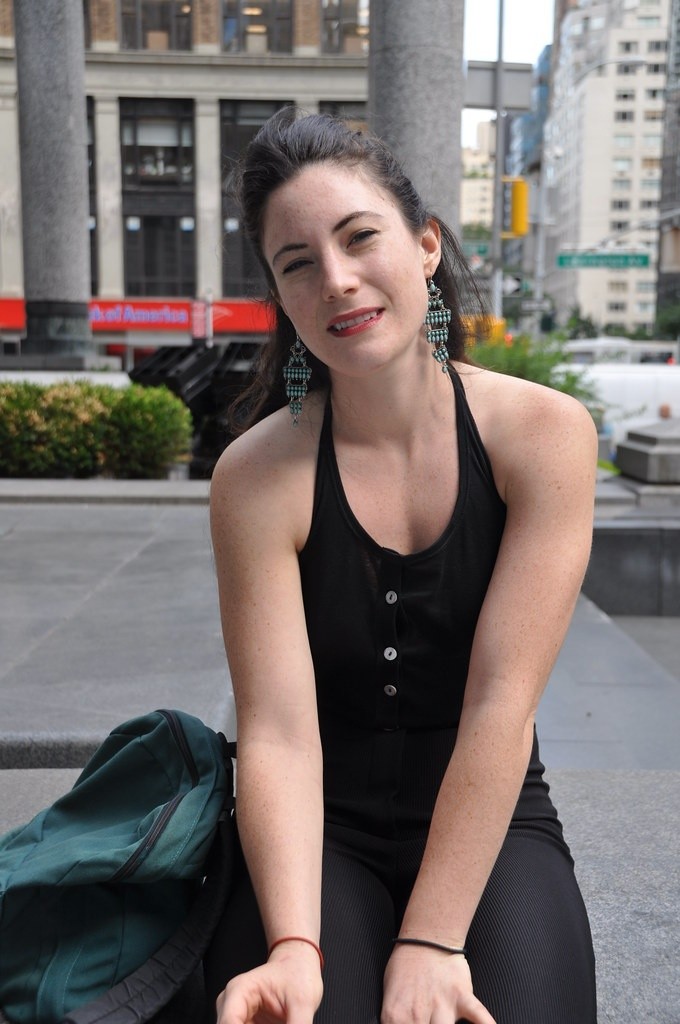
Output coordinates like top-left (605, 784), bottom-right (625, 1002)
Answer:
top-left (269, 936), bottom-right (326, 967)
top-left (393, 936), bottom-right (468, 956)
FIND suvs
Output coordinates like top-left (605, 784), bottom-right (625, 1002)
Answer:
top-left (126, 339), bottom-right (317, 481)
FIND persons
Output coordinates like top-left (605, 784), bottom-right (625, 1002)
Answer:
top-left (207, 105), bottom-right (600, 1023)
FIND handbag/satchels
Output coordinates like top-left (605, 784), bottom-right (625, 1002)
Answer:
top-left (0, 709), bottom-right (255, 1024)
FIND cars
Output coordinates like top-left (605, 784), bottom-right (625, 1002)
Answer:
top-left (559, 332), bottom-right (680, 366)
